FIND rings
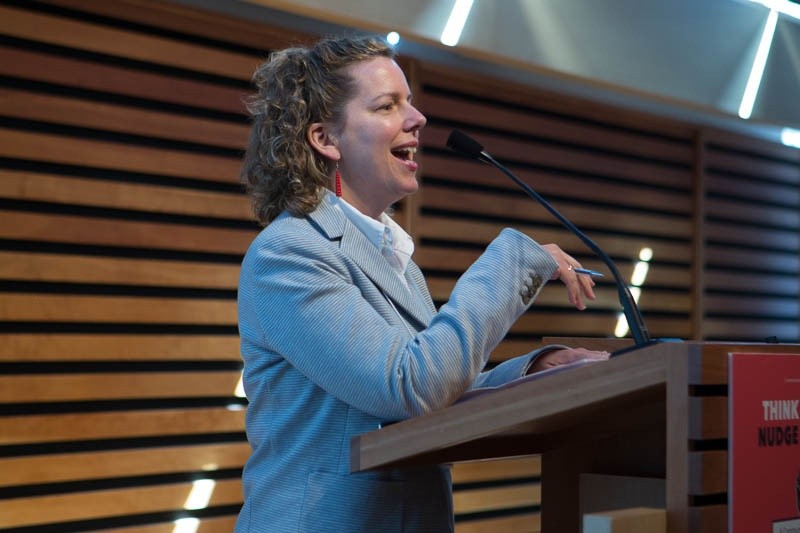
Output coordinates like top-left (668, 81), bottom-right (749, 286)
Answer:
top-left (568, 264), bottom-right (572, 270)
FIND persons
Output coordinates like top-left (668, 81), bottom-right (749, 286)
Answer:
top-left (232, 36), bottom-right (612, 533)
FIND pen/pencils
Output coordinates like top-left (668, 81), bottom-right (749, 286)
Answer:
top-left (573, 267), bottom-right (604, 277)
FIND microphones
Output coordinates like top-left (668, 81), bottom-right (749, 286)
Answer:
top-left (446, 129), bottom-right (685, 358)
